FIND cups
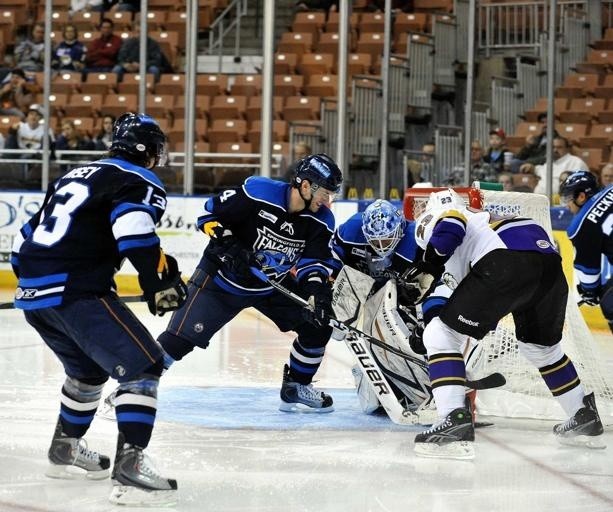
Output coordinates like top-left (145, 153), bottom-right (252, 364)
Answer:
top-left (504, 152), bottom-right (514, 166)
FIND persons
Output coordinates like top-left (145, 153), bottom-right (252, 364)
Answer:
top-left (10, 108), bottom-right (188, 492)
top-left (398, 190), bottom-right (609, 445)
top-left (101, 155), bottom-right (348, 421)
top-left (327, 198), bottom-right (438, 413)
top-left (558, 169), bottom-right (612, 353)
top-left (0, 17), bottom-right (176, 172)
top-left (467, 108), bottom-right (612, 203)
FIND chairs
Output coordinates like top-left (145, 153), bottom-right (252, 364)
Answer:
top-left (479, 0), bottom-right (613, 192)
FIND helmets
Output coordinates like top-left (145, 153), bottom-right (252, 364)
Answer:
top-left (109, 110), bottom-right (169, 168)
top-left (425, 188), bottom-right (460, 211)
top-left (557, 170), bottom-right (597, 208)
top-left (361, 198), bottom-right (406, 258)
top-left (292, 153), bottom-right (342, 205)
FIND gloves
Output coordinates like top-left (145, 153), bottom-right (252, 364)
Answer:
top-left (408, 325), bottom-right (427, 355)
top-left (137, 254), bottom-right (189, 316)
top-left (295, 269), bottom-right (335, 330)
top-left (580, 288), bottom-right (599, 306)
top-left (202, 238), bottom-right (262, 280)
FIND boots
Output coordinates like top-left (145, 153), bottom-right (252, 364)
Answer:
top-left (414, 407), bottom-right (474, 443)
top-left (280, 361), bottom-right (332, 408)
top-left (110, 429), bottom-right (177, 490)
top-left (48, 415), bottom-right (110, 472)
top-left (551, 392), bottom-right (603, 438)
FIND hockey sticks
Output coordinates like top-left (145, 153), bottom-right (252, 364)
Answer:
top-left (250, 267), bottom-right (506, 390)
top-left (344, 333), bottom-right (437, 425)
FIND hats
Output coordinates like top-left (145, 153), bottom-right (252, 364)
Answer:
top-left (29, 103), bottom-right (43, 119)
top-left (488, 127), bottom-right (505, 139)
